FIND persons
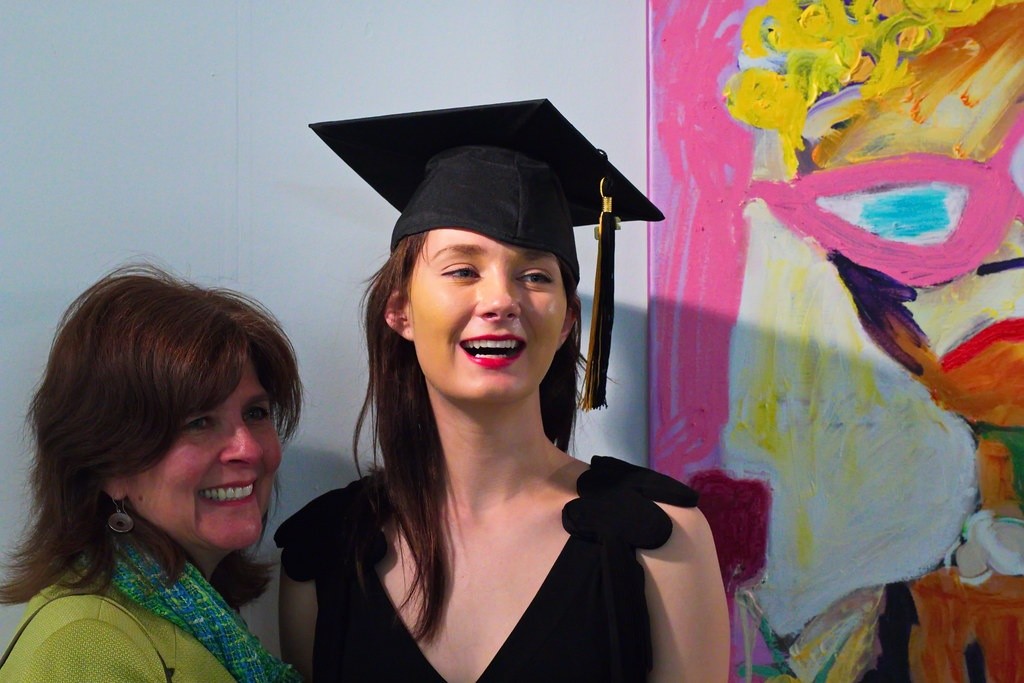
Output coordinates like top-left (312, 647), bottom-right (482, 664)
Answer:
top-left (0, 262), bottom-right (299, 683)
top-left (274, 97), bottom-right (736, 683)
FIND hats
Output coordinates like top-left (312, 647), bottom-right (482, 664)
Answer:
top-left (308, 98), bottom-right (665, 410)
